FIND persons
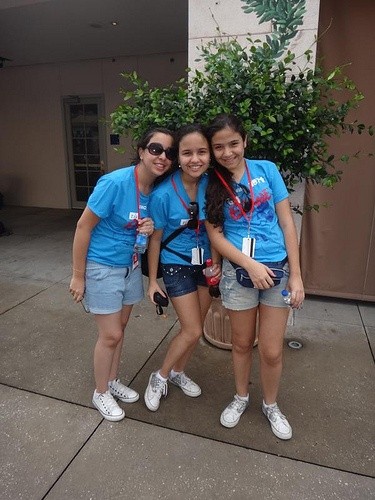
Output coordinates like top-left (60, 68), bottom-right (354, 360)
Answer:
top-left (197, 112), bottom-right (305, 439)
top-left (144, 122), bottom-right (214, 411)
top-left (69, 126), bottom-right (176, 421)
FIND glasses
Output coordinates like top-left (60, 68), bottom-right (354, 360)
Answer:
top-left (187, 201), bottom-right (199, 230)
top-left (237, 183), bottom-right (251, 213)
top-left (145, 143), bottom-right (176, 161)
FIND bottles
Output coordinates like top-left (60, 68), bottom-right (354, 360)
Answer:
top-left (132, 231), bottom-right (149, 254)
top-left (206, 259), bottom-right (223, 298)
top-left (281, 289), bottom-right (304, 311)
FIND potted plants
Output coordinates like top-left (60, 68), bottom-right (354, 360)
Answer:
top-left (109, 33), bottom-right (375, 351)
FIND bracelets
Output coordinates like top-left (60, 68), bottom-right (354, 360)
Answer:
top-left (72, 267), bottom-right (85, 273)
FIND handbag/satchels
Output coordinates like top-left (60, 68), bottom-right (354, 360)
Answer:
top-left (141, 241), bottom-right (162, 278)
top-left (229, 252), bottom-right (289, 287)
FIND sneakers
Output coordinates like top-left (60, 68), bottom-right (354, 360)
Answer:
top-left (92, 389), bottom-right (125, 421)
top-left (220, 393), bottom-right (249, 428)
top-left (169, 370), bottom-right (201, 397)
top-left (262, 400), bottom-right (292, 440)
top-left (109, 378), bottom-right (139, 403)
top-left (144, 370), bottom-right (167, 411)
